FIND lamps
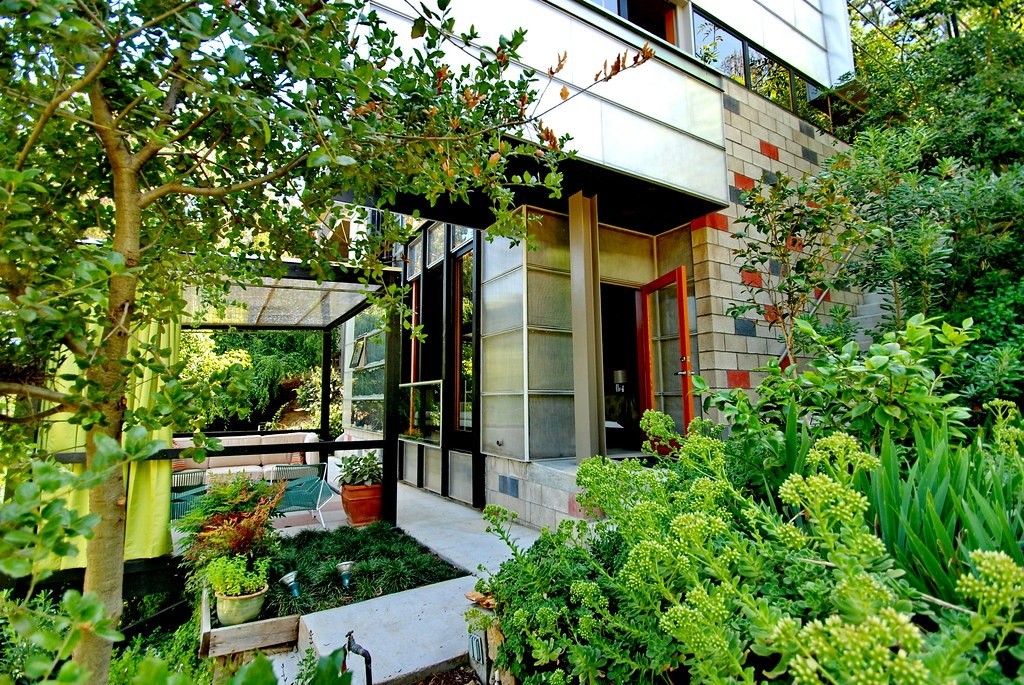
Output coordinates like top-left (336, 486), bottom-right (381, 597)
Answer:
top-left (613, 370), bottom-right (626, 393)
top-left (279, 570), bottom-right (300, 598)
top-left (335, 561), bottom-right (356, 589)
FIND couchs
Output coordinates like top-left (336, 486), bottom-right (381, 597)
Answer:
top-left (171, 431), bottom-right (320, 488)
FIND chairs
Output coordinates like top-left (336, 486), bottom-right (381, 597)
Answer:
top-left (268, 462), bottom-right (334, 530)
top-left (168, 470), bottom-right (209, 520)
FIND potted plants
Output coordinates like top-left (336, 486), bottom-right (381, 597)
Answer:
top-left (332, 449), bottom-right (383, 527)
top-left (163, 468), bottom-right (288, 625)
